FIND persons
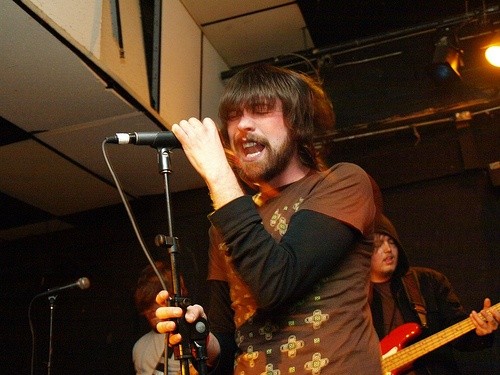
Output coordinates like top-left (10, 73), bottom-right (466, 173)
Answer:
top-left (155, 63), bottom-right (383, 375)
top-left (132, 260), bottom-right (220, 375)
top-left (369, 213), bottom-right (500, 375)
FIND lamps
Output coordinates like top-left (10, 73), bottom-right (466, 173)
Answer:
top-left (432, 18), bottom-right (464, 82)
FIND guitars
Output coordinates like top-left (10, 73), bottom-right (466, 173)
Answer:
top-left (379, 302), bottom-right (500, 375)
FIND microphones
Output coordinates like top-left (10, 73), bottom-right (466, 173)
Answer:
top-left (37, 277), bottom-right (90, 298)
top-left (106, 131), bottom-right (182, 149)
